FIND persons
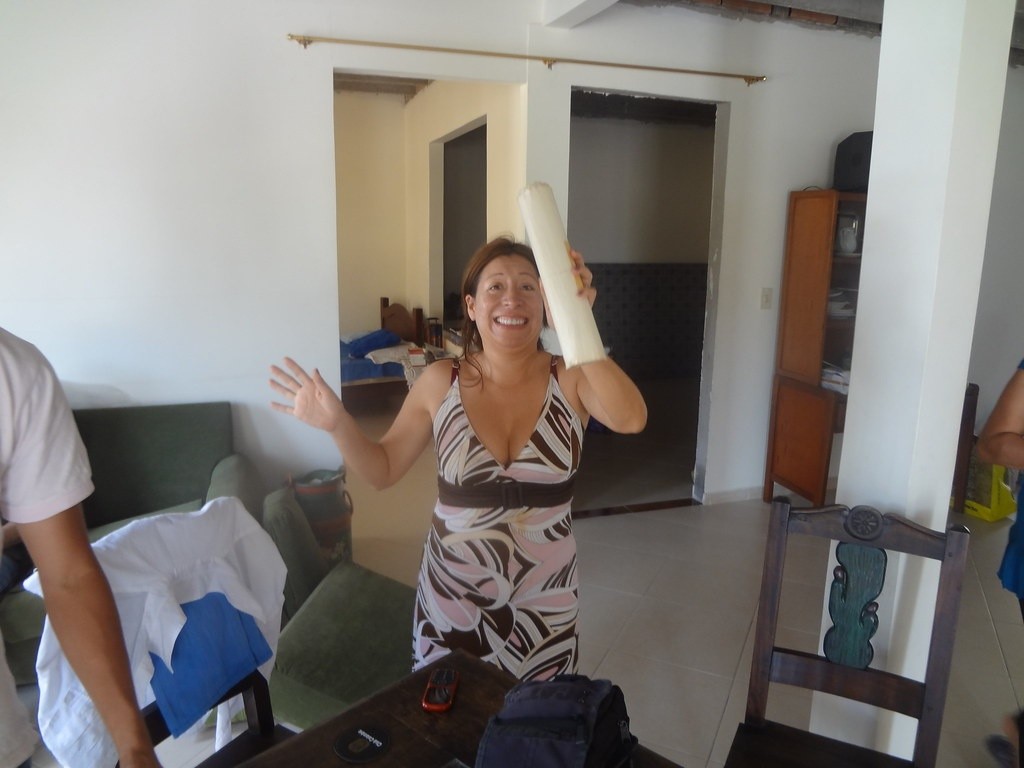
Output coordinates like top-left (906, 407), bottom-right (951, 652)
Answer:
top-left (971, 357), bottom-right (1023, 766)
top-left (0, 513), bottom-right (34, 603)
top-left (267, 237), bottom-right (648, 682)
top-left (0, 325), bottom-right (169, 768)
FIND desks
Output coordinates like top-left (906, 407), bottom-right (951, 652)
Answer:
top-left (218, 650), bottom-right (685, 768)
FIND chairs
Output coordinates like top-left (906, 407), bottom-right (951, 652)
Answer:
top-left (52, 506), bottom-right (297, 768)
top-left (724, 489), bottom-right (971, 768)
top-left (246, 480), bottom-right (419, 734)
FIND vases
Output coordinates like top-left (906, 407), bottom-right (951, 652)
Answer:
top-left (288, 468), bottom-right (355, 567)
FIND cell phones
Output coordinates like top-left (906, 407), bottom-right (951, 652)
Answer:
top-left (423, 669), bottom-right (460, 712)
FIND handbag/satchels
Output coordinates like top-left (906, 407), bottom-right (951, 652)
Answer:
top-left (474, 675), bottom-right (640, 768)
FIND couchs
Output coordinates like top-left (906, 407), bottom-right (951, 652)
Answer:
top-left (0, 400), bottom-right (267, 688)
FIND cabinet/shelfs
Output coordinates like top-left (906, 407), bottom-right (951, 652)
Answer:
top-left (762, 188), bottom-right (869, 507)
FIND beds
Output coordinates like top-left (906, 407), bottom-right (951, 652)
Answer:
top-left (339, 328), bottom-right (433, 408)
